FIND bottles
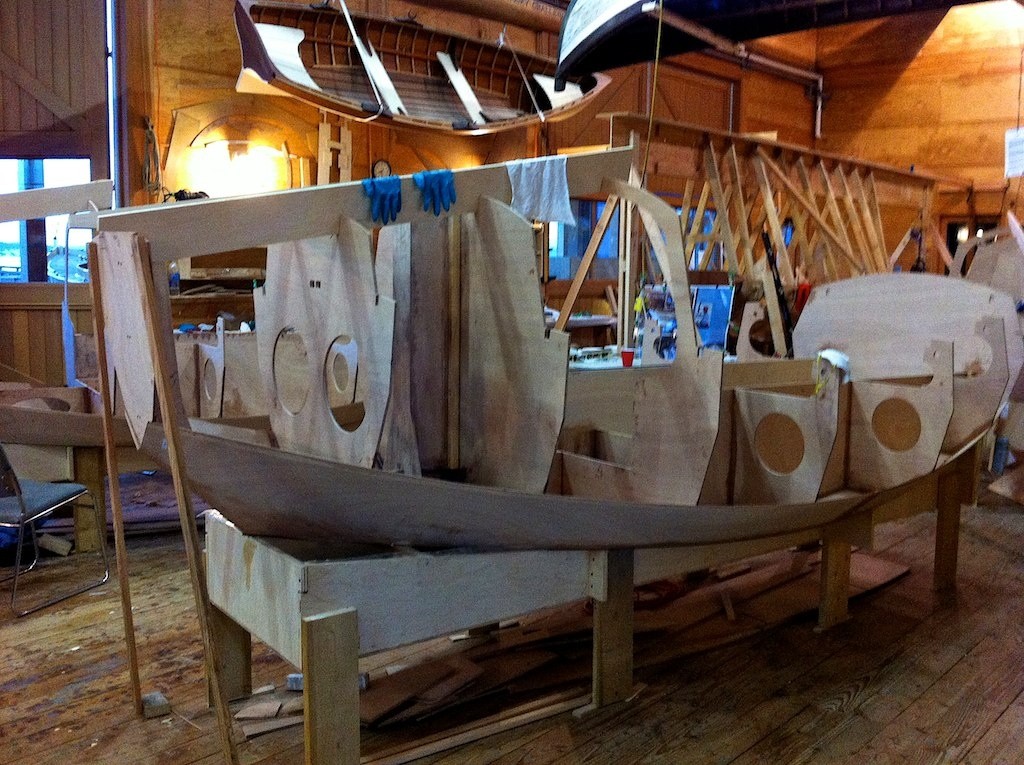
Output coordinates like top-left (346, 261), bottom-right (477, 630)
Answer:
top-left (993, 431), bottom-right (1010, 477)
top-left (168, 260), bottom-right (180, 296)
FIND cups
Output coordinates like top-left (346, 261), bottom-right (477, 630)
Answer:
top-left (621, 351), bottom-right (634, 367)
top-left (39, 533), bottom-right (72, 556)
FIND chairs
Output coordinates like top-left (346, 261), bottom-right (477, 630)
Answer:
top-left (0, 445), bottom-right (109, 617)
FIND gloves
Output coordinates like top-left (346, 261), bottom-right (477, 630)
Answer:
top-left (361, 172), bottom-right (403, 227)
top-left (414, 168), bottom-right (458, 217)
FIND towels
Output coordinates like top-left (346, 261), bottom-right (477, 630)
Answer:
top-left (505, 154), bottom-right (577, 229)
